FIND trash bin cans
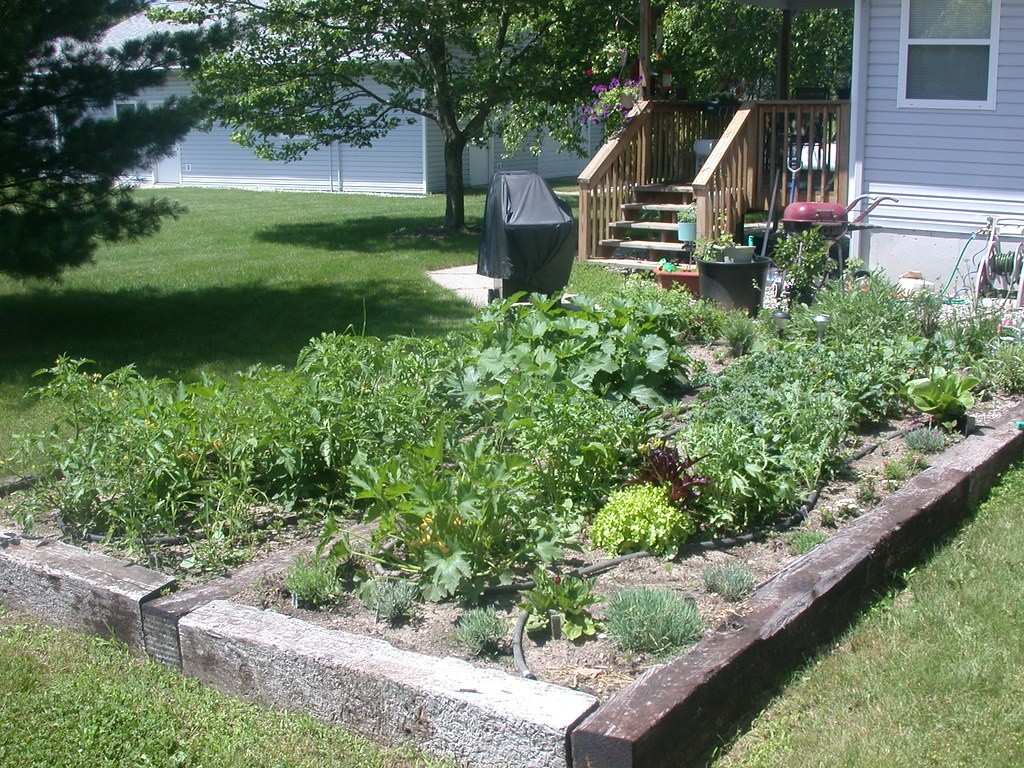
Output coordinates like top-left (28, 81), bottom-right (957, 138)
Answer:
top-left (488, 170), bottom-right (574, 307)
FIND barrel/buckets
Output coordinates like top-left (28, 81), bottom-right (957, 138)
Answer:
top-left (695, 256), bottom-right (771, 326)
